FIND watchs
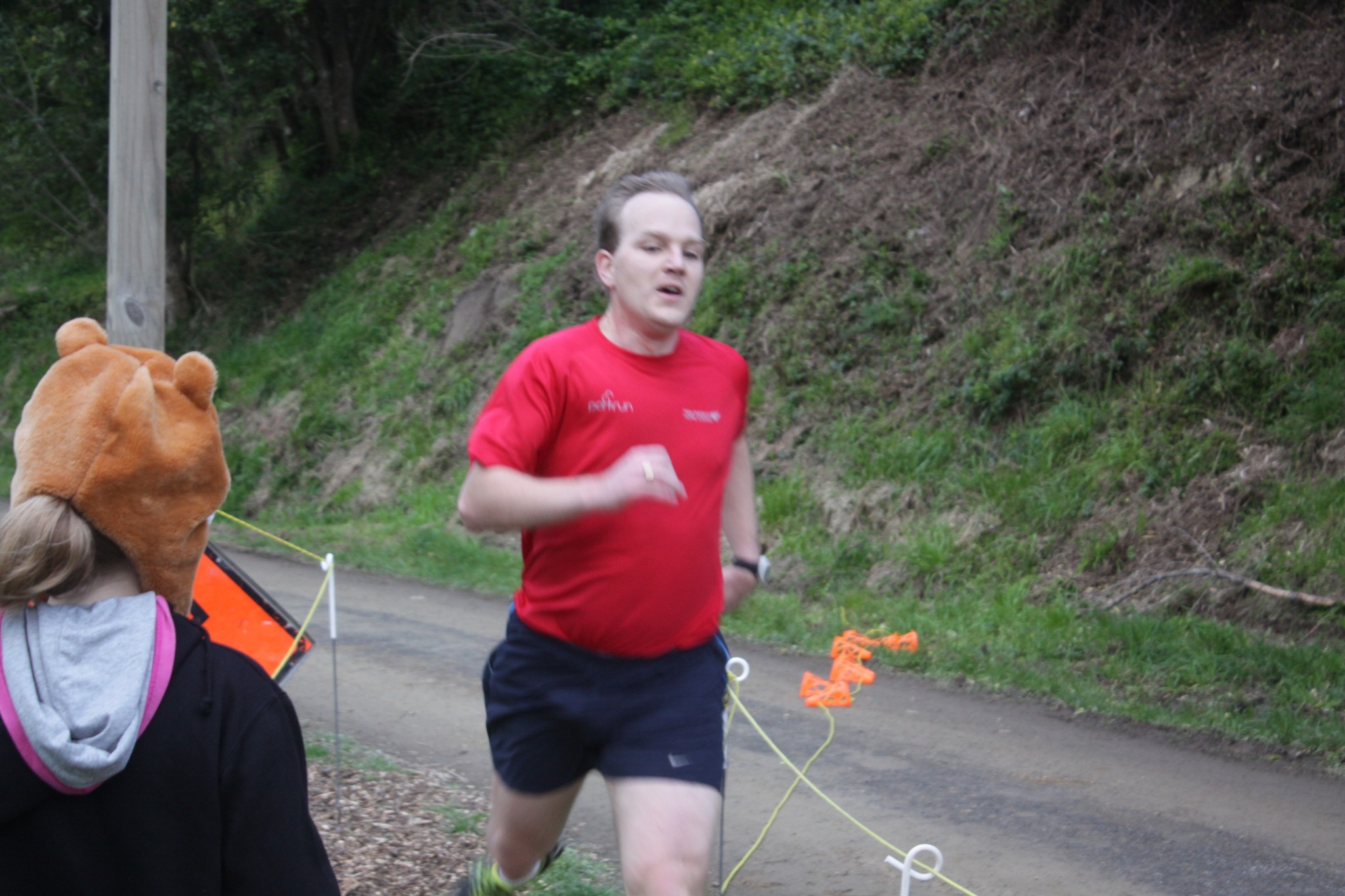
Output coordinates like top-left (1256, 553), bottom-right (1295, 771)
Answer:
top-left (730, 552), bottom-right (772, 586)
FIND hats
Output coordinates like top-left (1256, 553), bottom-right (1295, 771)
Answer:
top-left (10, 318), bottom-right (232, 619)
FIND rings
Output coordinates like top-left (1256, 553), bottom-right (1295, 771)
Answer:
top-left (641, 459), bottom-right (655, 482)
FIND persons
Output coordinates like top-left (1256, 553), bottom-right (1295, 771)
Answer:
top-left (452, 172), bottom-right (773, 896)
top-left (0, 316), bottom-right (344, 896)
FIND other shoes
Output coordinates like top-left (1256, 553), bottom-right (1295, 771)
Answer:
top-left (454, 840), bottom-right (565, 896)
